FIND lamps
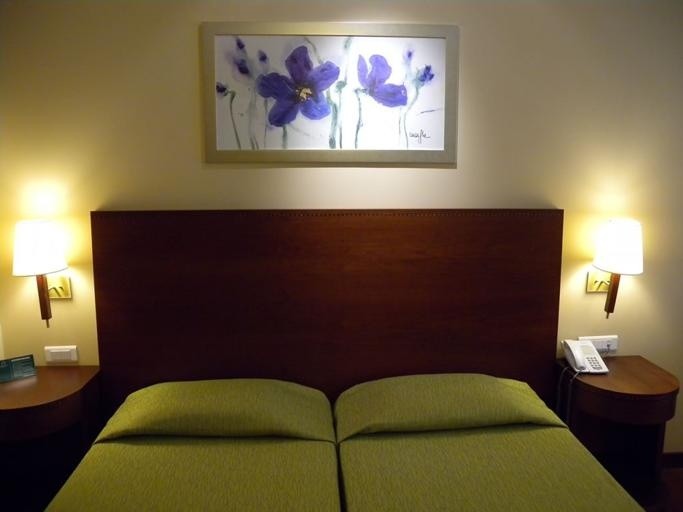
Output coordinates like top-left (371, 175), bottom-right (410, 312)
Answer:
top-left (582, 214), bottom-right (645, 322)
top-left (8, 217), bottom-right (75, 332)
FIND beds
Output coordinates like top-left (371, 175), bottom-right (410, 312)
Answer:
top-left (32, 209), bottom-right (585, 509)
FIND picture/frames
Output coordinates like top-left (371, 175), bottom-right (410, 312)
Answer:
top-left (197, 19), bottom-right (461, 171)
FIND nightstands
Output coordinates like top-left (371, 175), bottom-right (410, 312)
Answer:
top-left (565, 355), bottom-right (679, 504)
top-left (0, 359), bottom-right (98, 507)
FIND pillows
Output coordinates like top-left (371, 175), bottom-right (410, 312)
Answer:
top-left (93, 375), bottom-right (337, 446)
top-left (330, 369), bottom-right (566, 445)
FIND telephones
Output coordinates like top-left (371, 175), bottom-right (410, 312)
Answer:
top-left (560, 339), bottom-right (610, 375)
